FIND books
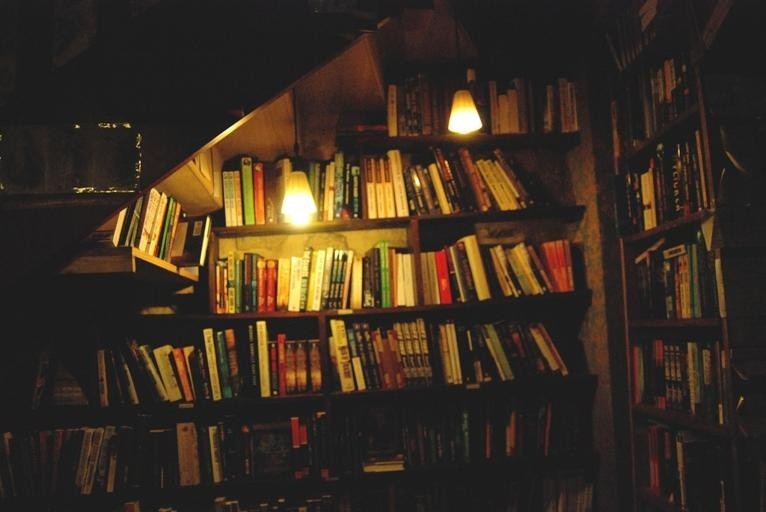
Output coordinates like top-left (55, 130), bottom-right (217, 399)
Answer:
top-left (386, 73), bottom-right (581, 136)
top-left (605, 0), bottom-right (740, 512)
top-left (215, 235), bottom-right (574, 312)
top-left (0, 316), bottom-right (594, 512)
top-left (91, 188), bottom-right (212, 268)
top-left (222, 147), bottom-right (536, 228)
